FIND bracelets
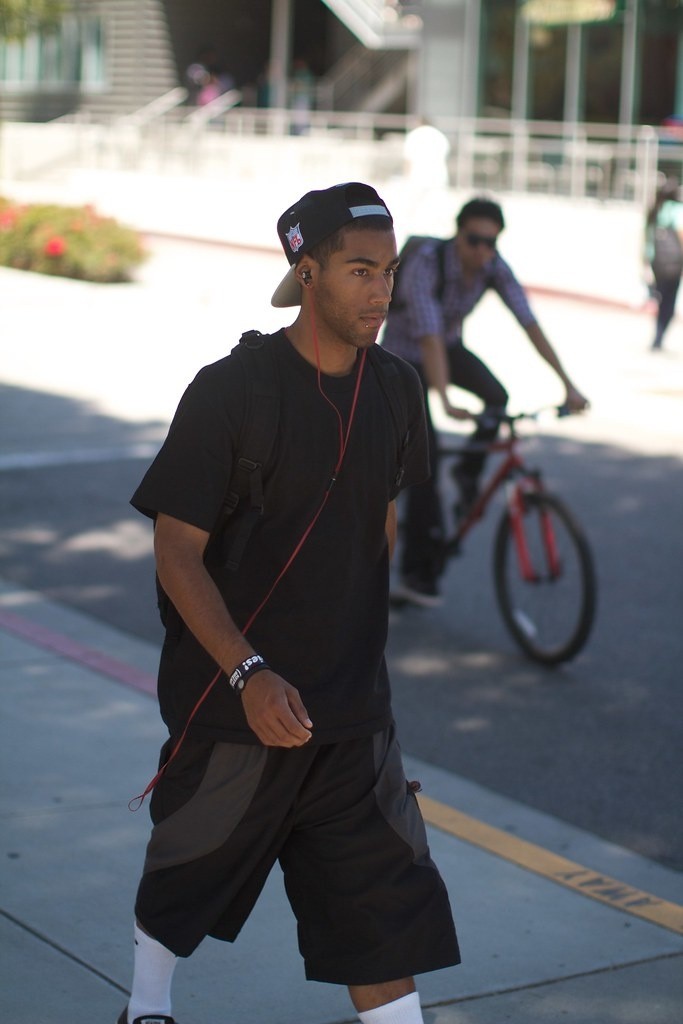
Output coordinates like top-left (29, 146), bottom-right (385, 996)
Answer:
top-left (228, 654), bottom-right (271, 696)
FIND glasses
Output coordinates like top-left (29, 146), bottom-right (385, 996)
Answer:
top-left (462, 230), bottom-right (498, 247)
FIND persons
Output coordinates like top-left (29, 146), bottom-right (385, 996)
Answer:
top-left (198, 75), bottom-right (221, 106)
top-left (378, 198), bottom-right (589, 606)
top-left (403, 117), bottom-right (451, 187)
top-left (640, 179), bottom-right (683, 350)
top-left (117, 182), bottom-right (463, 1024)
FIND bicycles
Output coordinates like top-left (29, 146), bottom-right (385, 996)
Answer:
top-left (395, 401), bottom-right (599, 667)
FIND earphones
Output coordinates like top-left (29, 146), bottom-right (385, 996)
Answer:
top-left (301, 271), bottom-right (313, 287)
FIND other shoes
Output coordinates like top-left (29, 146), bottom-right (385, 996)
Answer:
top-left (451, 463), bottom-right (478, 504)
top-left (399, 568), bottom-right (445, 606)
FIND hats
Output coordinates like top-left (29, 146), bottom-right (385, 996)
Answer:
top-left (270, 182), bottom-right (393, 308)
